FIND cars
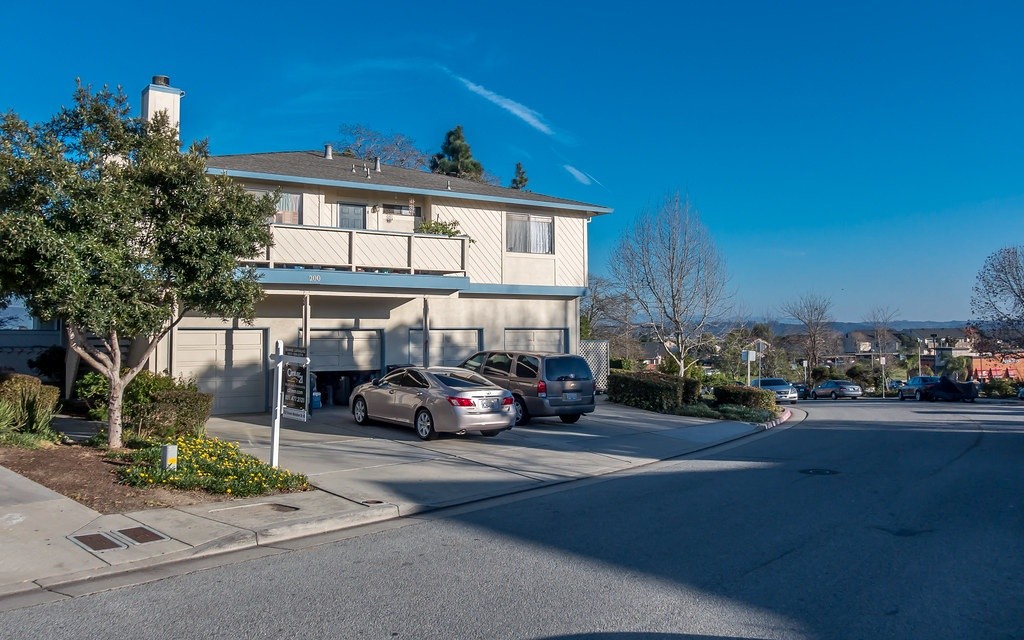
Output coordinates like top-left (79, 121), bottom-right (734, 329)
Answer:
top-left (898, 376), bottom-right (941, 402)
top-left (792, 383), bottom-right (808, 400)
top-left (889, 381), bottom-right (904, 390)
top-left (349, 367), bottom-right (516, 441)
top-left (810, 380), bottom-right (863, 400)
top-left (751, 377), bottom-right (798, 405)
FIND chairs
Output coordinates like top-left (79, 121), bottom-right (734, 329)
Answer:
top-left (493, 361), bottom-right (507, 373)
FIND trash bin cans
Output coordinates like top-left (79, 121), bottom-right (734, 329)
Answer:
top-left (313, 392), bottom-right (321, 409)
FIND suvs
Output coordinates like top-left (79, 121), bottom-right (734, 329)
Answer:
top-left (456, 349), bottom-right (598, 427)
